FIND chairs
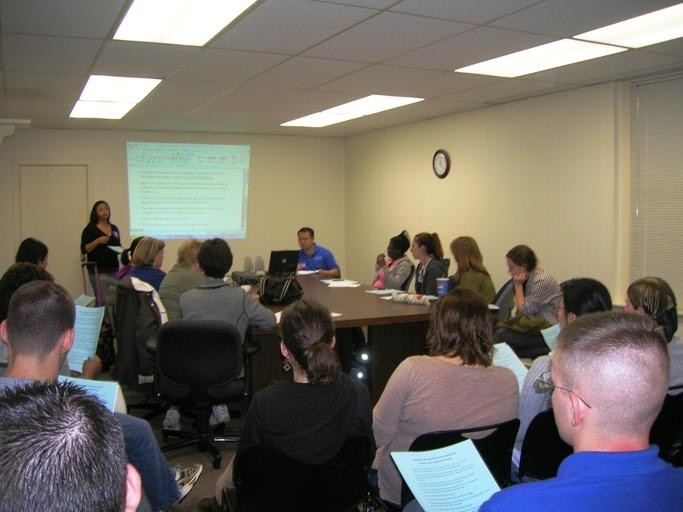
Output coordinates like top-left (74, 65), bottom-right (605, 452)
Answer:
top-left (518, 408), bottom-right (574, 480)
top-left (233, 425), bottom-right (369, 511)
top-left (648, 384), bottom-right (683, 468)
top-left (149, 318), bottom-right (260, 469)
top-left (112, 276), bottom-right (170, 420)
top-left (368, 417), bottom-right (520, 511)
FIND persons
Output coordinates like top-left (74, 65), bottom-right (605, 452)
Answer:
top-left (403, 309), bottom-right (682, 512)
top-left (621, 274), bottom-right (681, 404)
top-left (345, 280), bottom-right (521, 511)
top-left (0, 278), bottom-right (203, 512)
top-left (77, 200), bottom-right (121, 305)
top-left (509, 272), bottom-right (615, 484)
top-left (213, 297), bottom-right (374, 510)
top-left (1, 377), bottom-right (144, 512)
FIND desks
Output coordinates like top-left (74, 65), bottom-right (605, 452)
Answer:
top-left (234, 271), bottom-right (499, 403)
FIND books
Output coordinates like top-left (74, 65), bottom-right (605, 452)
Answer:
top-left (54, 374), bottom-right (120, 415)
top-left (389, 439), bottom-right (501, 511)
top-left (66, 304), bottom-right (106, 376)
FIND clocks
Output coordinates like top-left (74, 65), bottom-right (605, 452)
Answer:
top-left (432, 150), bottom-right (450, 179)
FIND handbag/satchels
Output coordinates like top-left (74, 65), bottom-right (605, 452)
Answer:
top-left (256, 270), bottom-right (305, 305)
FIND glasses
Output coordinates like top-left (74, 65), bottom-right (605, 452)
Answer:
top-left (536, 370), bottom-right (592, 408)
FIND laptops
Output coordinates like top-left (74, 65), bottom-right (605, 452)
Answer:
top-left (266, 250), bottom-right (300, 275)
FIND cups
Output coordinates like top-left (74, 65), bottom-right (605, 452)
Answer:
top-left (436, 278), bottom-right (449, 299)
top-left (240, 286), bottom-right (251, 293)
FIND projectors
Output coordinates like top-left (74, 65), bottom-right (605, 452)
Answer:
top-left (232, 271), bottom-right (258, 285)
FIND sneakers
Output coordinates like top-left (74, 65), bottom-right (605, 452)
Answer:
top-left (208, 403), bottom-right (230, 425)
top-left (162, 408), bottom-right (182, 431)
top-left (170, 462), bottom-right (204, 502)
top-left (197, 496), bottom-right (222, 511)
